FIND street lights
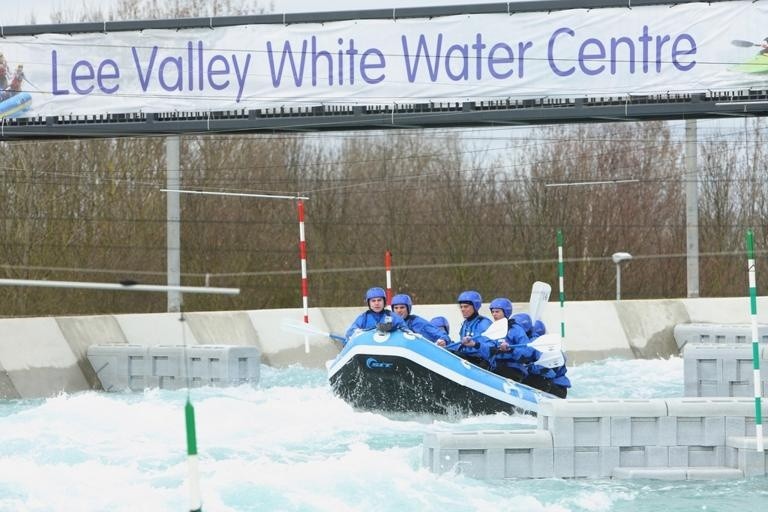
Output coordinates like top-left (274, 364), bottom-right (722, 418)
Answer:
top-left (611, 251), bottom-right (632, 299)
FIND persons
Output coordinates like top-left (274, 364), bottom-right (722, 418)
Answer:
top-left (391, 293), bottom-right (452, 347)
top-left (488, 298), bottom-right (529, 383)
top-left (345, 286), bottom-right (410, 340)
top-left (511, 313), bottom-right (556, 393)
top-left (534, 320), bottom-right (572, 399)
top-left (457, 290), bottom-right (499, 372)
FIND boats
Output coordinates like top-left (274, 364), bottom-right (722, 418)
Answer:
top-left (326, 321), bottom-right (563, 416)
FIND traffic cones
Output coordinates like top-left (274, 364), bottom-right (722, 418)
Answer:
top-left (296, 198), bottom-right (312, 356)
top-left (183, 399), bottom-right (202, 512)
top-left (555, 229), bottom-right (566, 339)
top-left (384, 250), bottom-right (393, 312)
top-left (745, 230), bottom-right (764, 455)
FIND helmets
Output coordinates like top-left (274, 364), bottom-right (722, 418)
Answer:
top-left (489, 298), bottom-right (512, 318)
top-left (431, 317), bottom-right (449, 334)
top-left (510, 313), bottom-right (532, 333)
top-left (533, 320), bottom-right (546, 335)
top-left (458, 291), bottom-right (482, 311)
top-left (365, 287), bottom-right (386, 303)
top-left (390, 294), bottom-right (412, 314)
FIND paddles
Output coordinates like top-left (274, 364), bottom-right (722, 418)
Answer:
top-left (282, 318), bottom-right (347, 342)
top-left (504, 333), bottom-right (563, 353)
top-left (524, 350), bottom-right (566, 369)
top-left (445, 318), bottom-right (508, 353)
top-left (529, 280), bottom-right (552, 331)
